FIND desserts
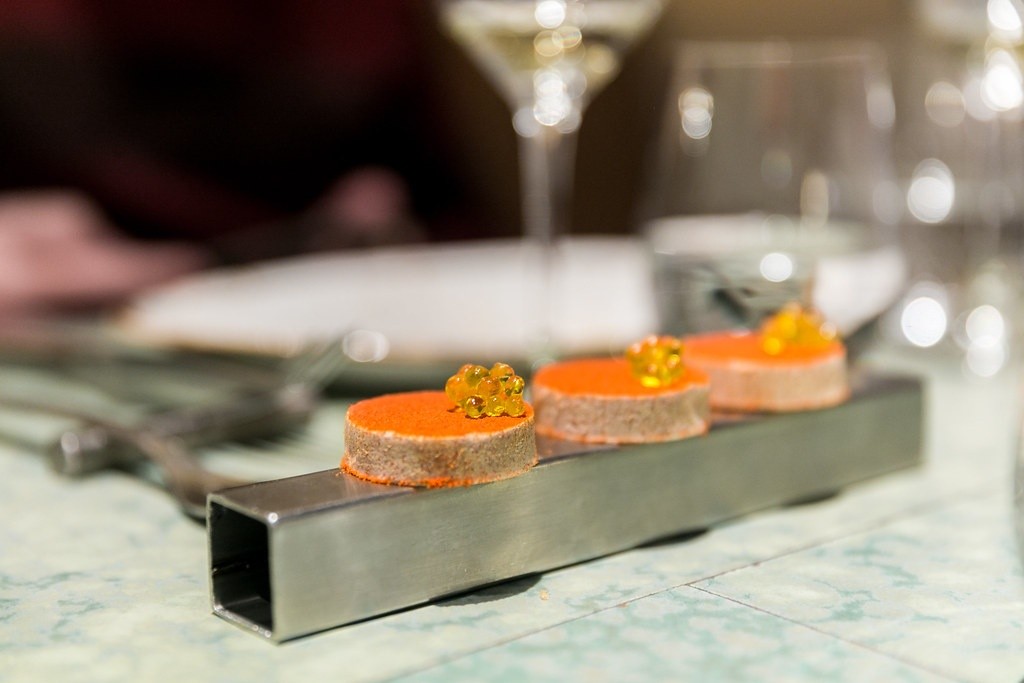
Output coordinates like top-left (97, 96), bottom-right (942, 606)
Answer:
top-left (343, 301), bottom-right (847, 487)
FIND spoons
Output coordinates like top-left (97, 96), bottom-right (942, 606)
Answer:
top-left (0, 367), bottom-right (293, 522)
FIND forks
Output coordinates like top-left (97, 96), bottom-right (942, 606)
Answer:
top-left (0, 334), bottom-right (368, 418)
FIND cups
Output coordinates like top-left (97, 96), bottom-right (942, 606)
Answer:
top-left (643, 38), bottom-right (911, 366)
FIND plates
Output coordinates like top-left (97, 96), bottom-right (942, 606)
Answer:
top-left (134, 233), bottom-right (907, 372)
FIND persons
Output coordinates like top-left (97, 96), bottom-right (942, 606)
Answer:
top-left (1, 0), bottom-right (685, 331)
